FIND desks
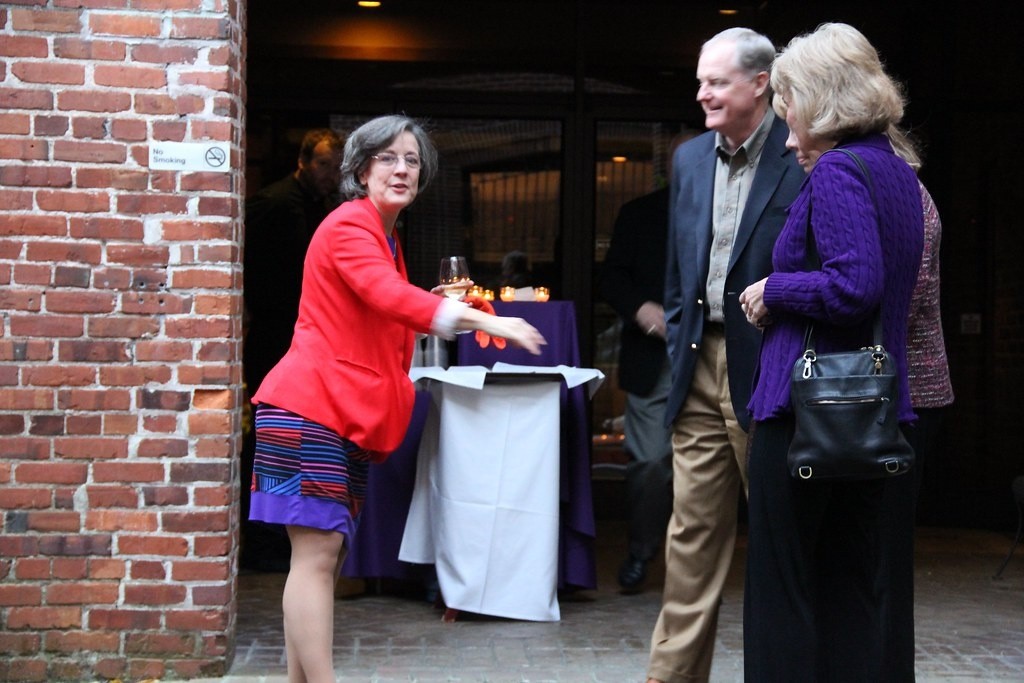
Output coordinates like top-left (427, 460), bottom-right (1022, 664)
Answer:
top-left (396, 362), bottom-right (607, 623)
top-left (346, 301), bottom-right (597, 600)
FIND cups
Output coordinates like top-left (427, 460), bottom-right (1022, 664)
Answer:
top-left (410, 338), bottom-right (423, 368)
top-left (424, 335), bottom-right (448, 369)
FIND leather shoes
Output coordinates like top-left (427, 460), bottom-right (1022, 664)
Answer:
top-left (616, 544), bottom-right (662, 592)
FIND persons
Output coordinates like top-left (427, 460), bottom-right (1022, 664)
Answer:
top-left (774, 92), bottom-right (954, 447)
top-left (645, 26), bottom-right (807, 683)
top-left (601, 130), bottom-right (704, 593)
top-left (247, 114), bottom-right (547, 683)
top-left (239, 128), bottom-right (343, 574)
top-left (742, 23), bottom-right (926, 683)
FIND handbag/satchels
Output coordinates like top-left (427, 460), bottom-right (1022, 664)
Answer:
top-left (786, 148), bottom-right (917, 487)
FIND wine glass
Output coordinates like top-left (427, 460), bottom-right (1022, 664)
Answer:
top-left (439, 256), bottom-right (473, 334)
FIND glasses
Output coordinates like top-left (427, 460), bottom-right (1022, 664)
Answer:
top-left (371, 153), bottom-right (426, 171)
